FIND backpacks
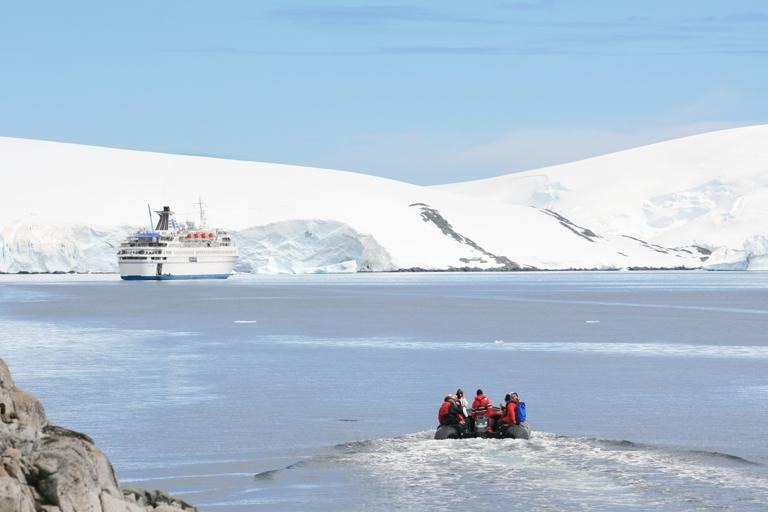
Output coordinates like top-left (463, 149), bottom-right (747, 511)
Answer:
top-left (517, 402), bottom-right (526, 422)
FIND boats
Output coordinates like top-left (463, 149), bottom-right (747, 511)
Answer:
top-left (116, 195), bottom-right (240, 280)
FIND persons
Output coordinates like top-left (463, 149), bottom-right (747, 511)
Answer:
top-left (438, 387), bottom-right (527, 438)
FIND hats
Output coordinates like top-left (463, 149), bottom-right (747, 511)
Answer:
top-left (457, 389), bottom-right (463, 399)
top-left (477, 389), bottom-right (482, 395)
top-left (504, 394), bottom-right (510, 401)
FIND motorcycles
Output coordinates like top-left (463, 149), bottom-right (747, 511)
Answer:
top-left (435, 405), bottom-right (531, 441)
